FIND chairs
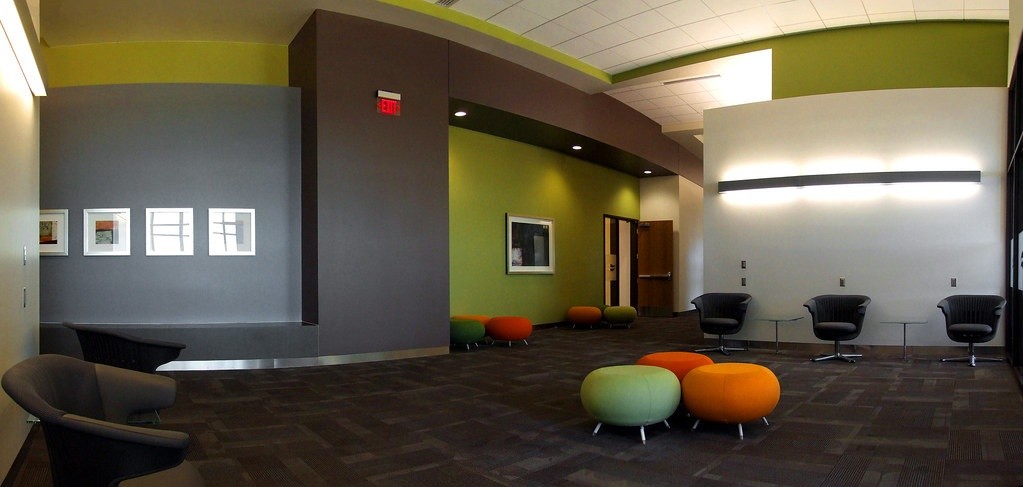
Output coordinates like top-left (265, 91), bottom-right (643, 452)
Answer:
top-left (689, 293), bottom-right (752, 355)
top-left (805, 294), bottom-right (870, 362)
top-left (938, 294), bottom-right (1007, 366)
top-left (63, 322), bottom-right (187, 374)
top-left (0, 352), bottom-right (206, 486)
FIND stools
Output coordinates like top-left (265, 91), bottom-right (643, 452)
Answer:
top-left (580, 366), bottom-right (682, 444)
top-left (682, 364), bottom-right (779, 433)
top-left (449, 315), bottom-right (532, 349)
top-left (638, 352), bottom-right (715, 381)
top-left (568, 304), bottom-right (637, 329)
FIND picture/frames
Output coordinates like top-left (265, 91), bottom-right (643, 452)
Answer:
top-left (81, 209), bottom-right (130, 257)
top-left (145, 208), bottom-right (194, 257)
top-left (208, 208), bottom-right (256, 256)
top-left (39, 210), bottom-right (69, 257)
top-left (505, 212), bottom-right (556, 275)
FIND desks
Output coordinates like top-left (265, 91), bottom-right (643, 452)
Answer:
top-left (881, 319), bottom-right (928, 361)
top-left (755, 314), bottom-right (804, 356)
